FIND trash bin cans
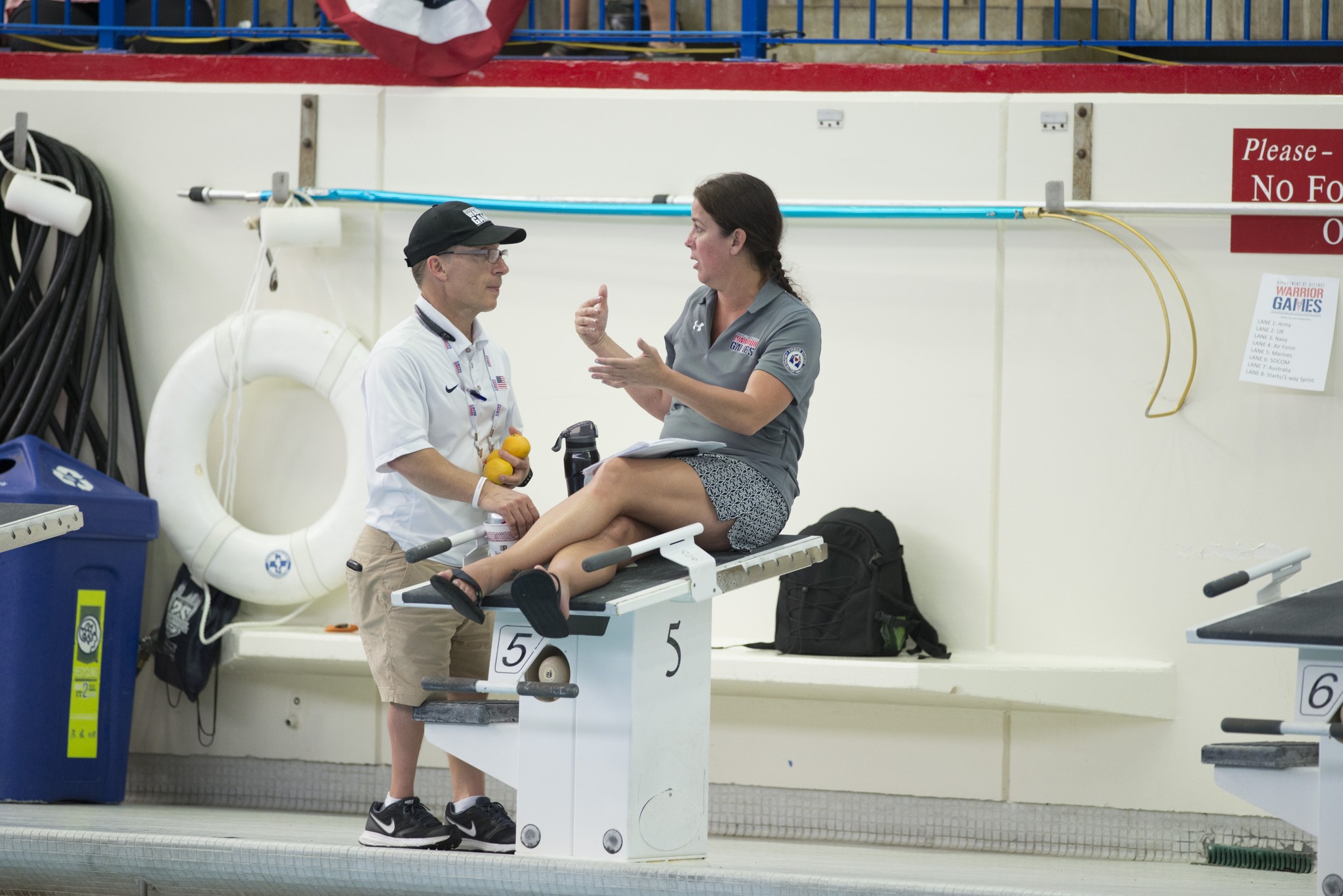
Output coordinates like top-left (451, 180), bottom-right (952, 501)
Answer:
top-left (0, 435), bottom-right (158, 806)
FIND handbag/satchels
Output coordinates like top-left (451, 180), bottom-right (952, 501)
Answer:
top-left (155, 564), bottom-right (240, 747)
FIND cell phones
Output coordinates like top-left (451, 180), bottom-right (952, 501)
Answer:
top-left (664, 448), bottom-right (700, 458)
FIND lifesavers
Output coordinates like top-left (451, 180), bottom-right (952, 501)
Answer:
top-left (144, 309), bottom-right (373, 608)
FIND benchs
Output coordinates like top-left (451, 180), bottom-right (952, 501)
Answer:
top-left (222, 624), bottom-right (1168, 721)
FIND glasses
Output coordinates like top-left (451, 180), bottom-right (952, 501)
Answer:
top-left (435, 249), bottom-right (508, 264)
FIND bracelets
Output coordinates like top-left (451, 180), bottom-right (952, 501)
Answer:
top-left (472, 476), bottom-right (487, 508)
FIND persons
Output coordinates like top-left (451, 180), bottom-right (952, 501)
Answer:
top-left (428, 172), bottom-right (822, 638)
top-left (344, 202), bottom-right (541, 852)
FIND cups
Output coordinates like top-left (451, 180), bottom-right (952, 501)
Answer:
top-left (483, 520), bottom-right (521, 557)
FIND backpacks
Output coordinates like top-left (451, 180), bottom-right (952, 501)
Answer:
top-left (774, 508), bottom-right (951, 659)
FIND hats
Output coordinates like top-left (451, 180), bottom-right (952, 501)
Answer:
top-left (404, 201), bottom-right (526, 267)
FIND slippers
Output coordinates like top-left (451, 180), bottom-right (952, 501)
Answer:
top-left (511, 568), bottom-right (569, 638)
top-left (430, 569), bottom-right (485, 625)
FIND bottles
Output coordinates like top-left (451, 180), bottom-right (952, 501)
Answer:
top-left (551, 420), bottom-right (600, 497)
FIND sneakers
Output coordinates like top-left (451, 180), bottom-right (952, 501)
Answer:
top-left (444, 797), bottom-right (516, 852)
top-left (359, 797), bottom-right (462, 849)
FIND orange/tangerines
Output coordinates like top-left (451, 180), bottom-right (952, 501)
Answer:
top-left (483, 434), bottom-right (531, 485)
